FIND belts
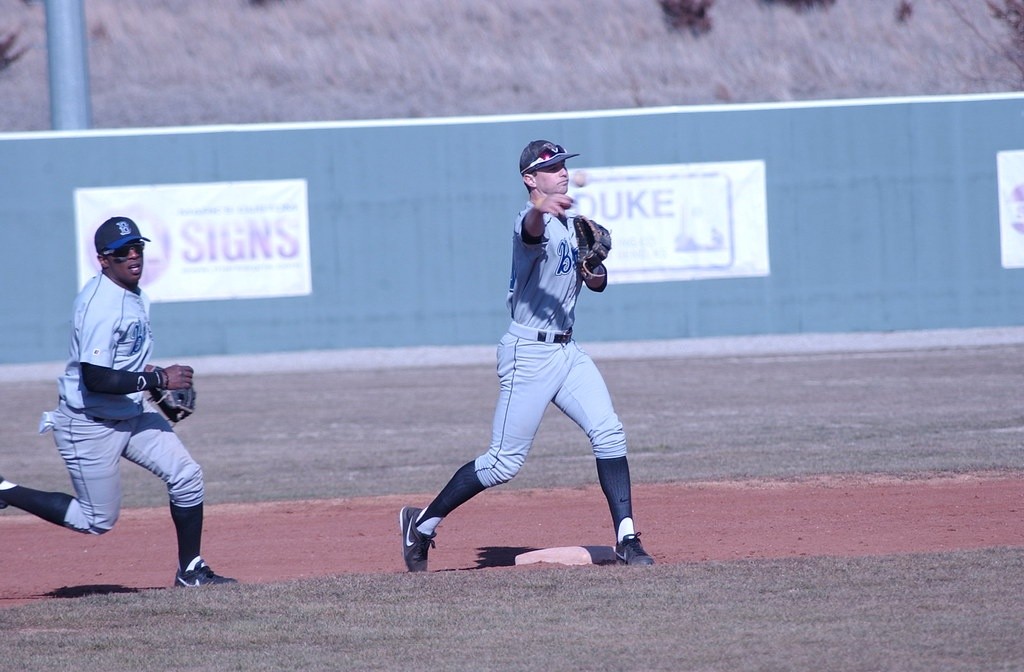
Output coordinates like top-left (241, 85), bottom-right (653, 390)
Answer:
top-left (59, 396), bottom-right (105, 422)
top-left (538, 328), bottom-right (572, 343)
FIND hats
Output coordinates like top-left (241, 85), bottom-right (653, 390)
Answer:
top-left (519, 140), bottom-right (580, 176)
top-left (94, 217), bottom-right (151, 254)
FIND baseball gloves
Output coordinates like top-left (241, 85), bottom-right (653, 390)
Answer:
top-left (145, 364), bottom-right (195, 421)
top-left (573, 214), bottom-right (611, 277)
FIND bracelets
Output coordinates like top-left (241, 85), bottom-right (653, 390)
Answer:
top-left (160, 370), bottom-right (169, 389)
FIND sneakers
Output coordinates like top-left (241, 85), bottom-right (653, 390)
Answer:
top-left (0, 475), bottom-right (8, 509)
top-left (174, 559), bottom-right (238, 587)
top-left (616, 532), bottom-right (654, 566)
top-left (399, 505), bottom-right (437, 572)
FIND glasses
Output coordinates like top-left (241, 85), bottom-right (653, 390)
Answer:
top-left (536, 143), bottom-right (567, 155)
top-left (98, 241), bottom-right (145, 257)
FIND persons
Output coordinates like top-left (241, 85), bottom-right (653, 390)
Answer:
top-left (0, 217), bottom-right (236, 589)
top-left (399, 138), bottom-right (654, 569)
